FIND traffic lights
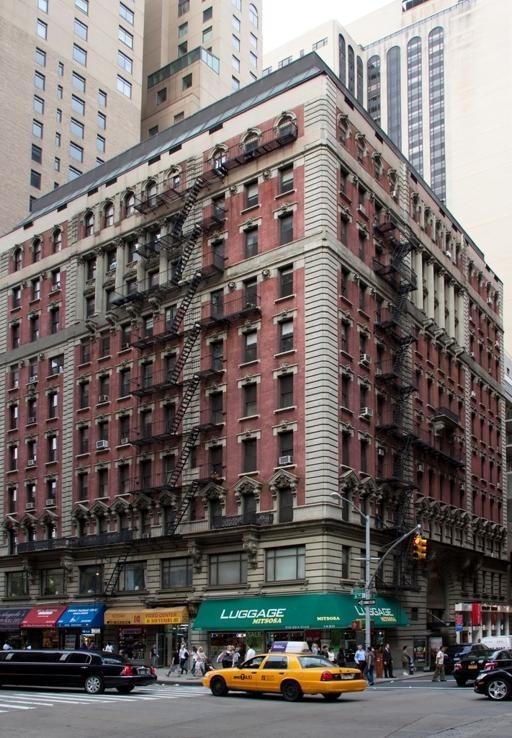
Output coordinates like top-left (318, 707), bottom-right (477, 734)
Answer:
top-left (411, 537), bottom-right (421, 561)
top-left (419, 538), bottom-right (427, 559)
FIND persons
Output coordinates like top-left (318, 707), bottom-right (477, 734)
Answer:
top-left (3, 640), bottom-right (12, 650)
top-left (383, 643), bottom-right (396, 678)
top-left (23, 640), bottom-right (31, 650)
top-left (80, 641), bottom-right (256, 678)
top-left (311, 644), bottom-right (375, 685)
top-left (401, 646), bottom-right (410, 675)
top-left (432, 646), bottom-right (446, 682)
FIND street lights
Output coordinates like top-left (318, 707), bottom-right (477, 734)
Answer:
top-left (329, 490), bottom-right (372, 652)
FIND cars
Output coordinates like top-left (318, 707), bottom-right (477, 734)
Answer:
top-left (472, 666), bottom-right (512, 699)
top-left (201, 640), bottom-right (369, 702)
top-left (453, 649), bottom-right (512, 686)
top-left (0, 647), bottom-right (157, 698)
top-left (442, 643), bottom-right (490, 670)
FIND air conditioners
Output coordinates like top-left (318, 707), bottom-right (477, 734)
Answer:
top-left (361, 353), bottom-right (370, 364)
top-left (110, 262), bottom-right (117, 269)
top-left (278, 455), bottom-right (293, 464)
top-left (487, 296), bottom-right (493, 304)
top-left (46, 499), bottom-right (55, 505)
top-left (30, 376), bottom-right (37, 382)
top-left (417, 465), bottom-right (424, 472)
top-left (28, 460), bottom-right (35, 466)
top-left (360, 407), bottom-right (373, 417)
top-left (25, 502), bottom-right (34, 509)
top-left (392, 191), bottom-right (398, 198)
top-left (96, 440), bottom-right (108, 448)
top-left (120, 438), bottom-right (128, 444)
top-left (379, 449), bottom-right (384, 456)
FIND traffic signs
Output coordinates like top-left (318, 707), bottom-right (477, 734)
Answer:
top-left (354, 593), bottom-right (365, 599)
top-left (350, 588), bottom-right (365, 595)
top-left (357, 599), bottom-right (376, 606)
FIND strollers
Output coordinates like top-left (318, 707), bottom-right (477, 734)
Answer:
top-left (199, 657), bottom-right (215, 675)
